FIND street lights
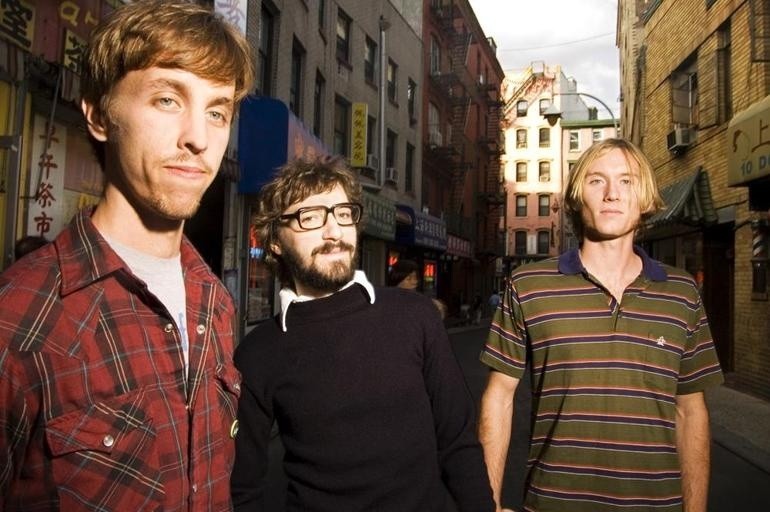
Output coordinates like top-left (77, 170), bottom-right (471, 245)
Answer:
top-left (544, 91), bottom-right (618, 136)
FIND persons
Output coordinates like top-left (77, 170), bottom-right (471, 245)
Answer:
top-left (488, 289), bottom-right (501, 325)
top-left (472, 289), bottom-right (483, 323)
top-left (0, 1), bottom-right (256, 512)
top-left (234, 153), bottom-right (493, 512)
top-left (16, 238), bottom-right (50, 258)
top-left (477, 138), bottom-right (726, 512)
top-left (384, 259), bottom-right (445, 322)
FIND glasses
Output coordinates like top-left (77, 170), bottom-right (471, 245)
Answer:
top-left (278, 202), bottom-right (362, 231)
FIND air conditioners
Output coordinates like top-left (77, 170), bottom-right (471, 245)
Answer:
top-left (666, 127), bottom-right (690, 151)
top-left (367, 154), bottom-right (400, 184)
